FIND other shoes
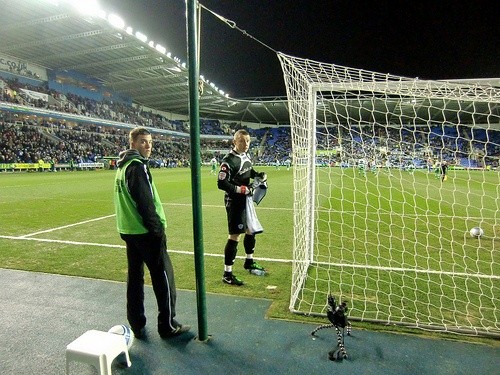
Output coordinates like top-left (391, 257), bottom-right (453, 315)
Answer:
top-left (177, 325), bottom-right (191, 335)
top-left (135, 326), bottom-right (145, 338)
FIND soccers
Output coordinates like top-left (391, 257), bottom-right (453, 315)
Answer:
top-left (108, 324), bottom-right (134, 350)
top-left (469, 227), bottom-right (483, 238)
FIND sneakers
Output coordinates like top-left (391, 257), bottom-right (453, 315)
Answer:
top-left (244, 262), bottom-right (265, 275)
top-left (222, 276), bottom-right (243, 285)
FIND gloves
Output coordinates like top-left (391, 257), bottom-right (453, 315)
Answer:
top-left (236, 186), bottom-right (250, 194)
top-left (255, 172), bottom-right (265, 179)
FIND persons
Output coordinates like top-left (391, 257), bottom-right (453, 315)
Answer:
top-left (113, 127), bottom-right (191, 339)
top-left (0, 75), bottom-right (500, 182)
top-left (217, 130), bottom-right (268, 285)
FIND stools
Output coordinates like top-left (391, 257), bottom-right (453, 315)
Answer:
top-left (65, 329), bottom-right (132, 375)
